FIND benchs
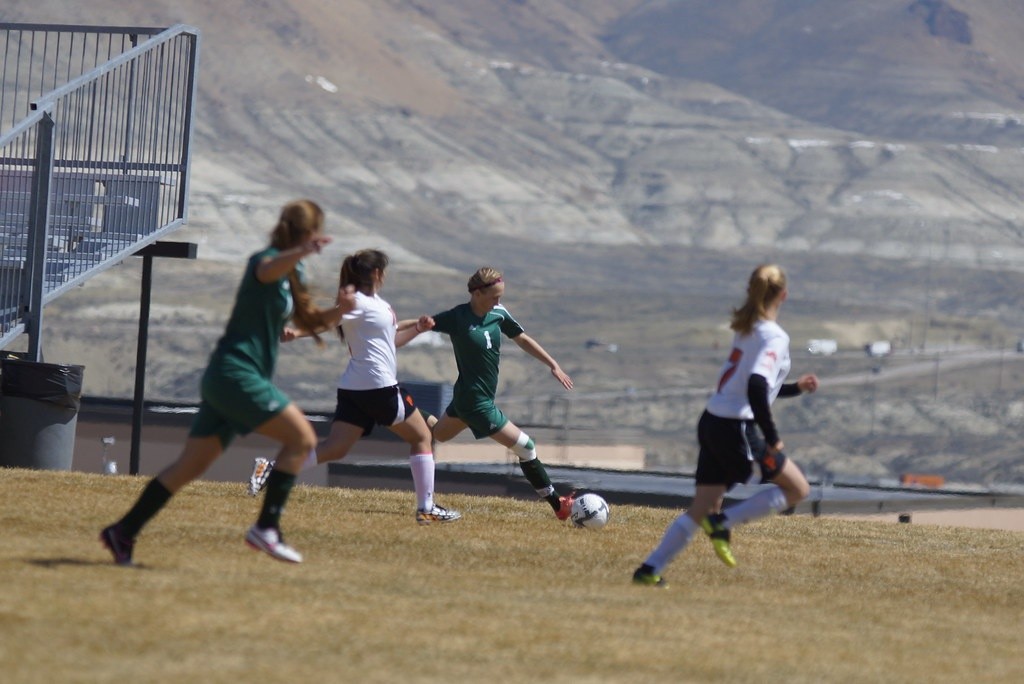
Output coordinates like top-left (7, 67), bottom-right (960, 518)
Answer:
top-left (0, 170), bottom-right (176, 306)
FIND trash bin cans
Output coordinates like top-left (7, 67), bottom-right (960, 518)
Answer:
top-left (402, 380), bottom-right (454, 419)
top-left (0, 358), bottom-right (86, 472)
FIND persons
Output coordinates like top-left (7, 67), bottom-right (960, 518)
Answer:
top-left (278, 248), bottom-right (462, 524)
top-left (631, 265), bottom-right (820, 589)
top-left (393, 266), bottom-right (577, 521)
top-left (97, 198), bottom-right (357, 572)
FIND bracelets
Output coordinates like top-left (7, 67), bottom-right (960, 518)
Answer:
top-left (416, 324), bottom-right (422, 334)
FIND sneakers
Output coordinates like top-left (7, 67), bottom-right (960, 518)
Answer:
top-left (249, 457), bottom-right (276, 496)
top-left (632, 563), bottom-right (671, 590)
top-left (700, 512), bottom-right (737, 567)
top-left (244, 523), bottom-right (304, 563)
top-left (417, 504), bottom-right (461, 525)
top-left (556, 491), bottom-right (576, 521)
top-left (100, 525), bottom-right (137, 565)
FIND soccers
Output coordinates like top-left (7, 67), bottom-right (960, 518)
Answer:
top-left (571, 492), bottom-right (609, 530)
